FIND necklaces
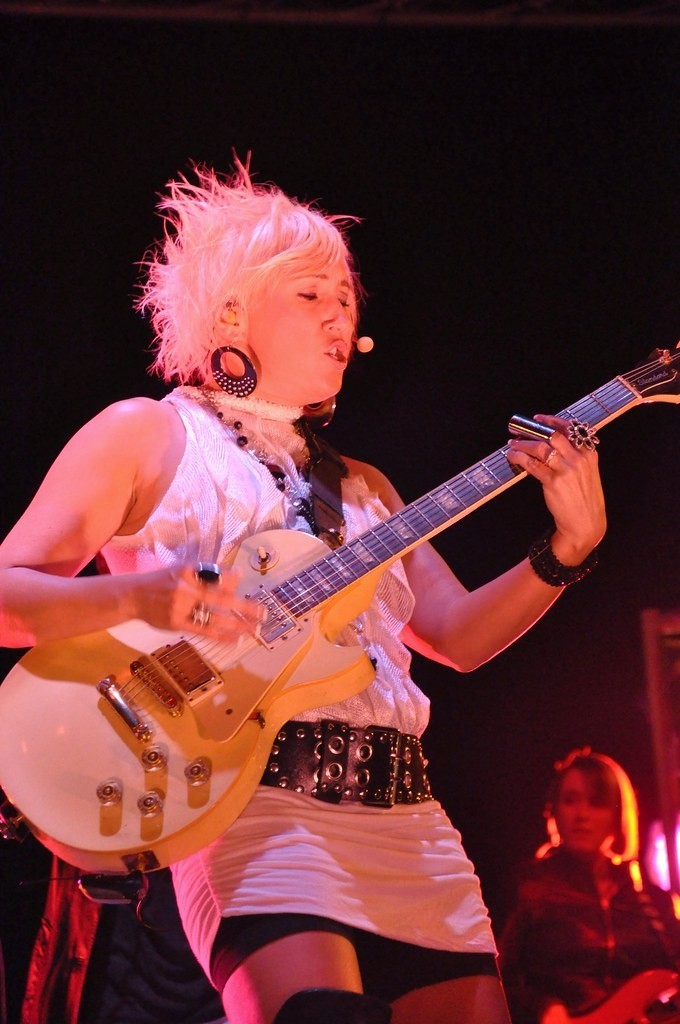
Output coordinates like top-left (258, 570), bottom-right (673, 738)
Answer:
top-left (210, 409), bottom-right (316, 536)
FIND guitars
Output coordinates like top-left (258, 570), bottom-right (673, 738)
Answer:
top-left (540, 968), bottom-right (680, 1024)
top-left (0, 342), bottom-right (680, 880)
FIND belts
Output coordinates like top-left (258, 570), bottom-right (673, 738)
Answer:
top-left (259, 718), bottom-right (433, 809)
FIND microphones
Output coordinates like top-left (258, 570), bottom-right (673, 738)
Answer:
top-left (352, 336), bottom-right (374, 353)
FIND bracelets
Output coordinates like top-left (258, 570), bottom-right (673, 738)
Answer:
top-left (529, 527), bottom-right (599, 587)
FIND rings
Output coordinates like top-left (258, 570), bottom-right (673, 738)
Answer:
top-left (187, 601), bottom-right (212, 630)
top-left (545, 450), bottom-right (558, 463)
top-left (567, 419), bottom-right (600, 452)
top-left (193, 561), bottom-right (221, 588)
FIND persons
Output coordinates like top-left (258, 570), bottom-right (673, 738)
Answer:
top-left (497, 745), bottom-right (680, 1024)
top-left (0, 149), bottom-right (608, 1024)
